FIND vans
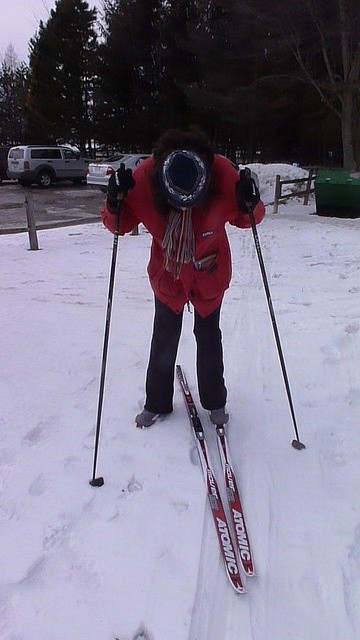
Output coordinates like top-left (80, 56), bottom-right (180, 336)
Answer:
top-left (7, 144), bottom-right (88, 189)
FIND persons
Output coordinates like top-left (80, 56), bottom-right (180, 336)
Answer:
top-left (103, 130), bottom-right (265, 426)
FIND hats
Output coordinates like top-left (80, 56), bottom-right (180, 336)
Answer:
top-left (157, 136), bottom-right (211, 209)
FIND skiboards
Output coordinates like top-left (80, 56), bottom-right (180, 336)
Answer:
top-left (175, 364), bottom-right (254, 595)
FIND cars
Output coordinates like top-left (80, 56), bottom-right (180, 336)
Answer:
top-left (85, 152), bottom-right (153, 193)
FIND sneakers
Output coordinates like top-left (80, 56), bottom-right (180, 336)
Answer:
top-left (206, 406), bottom-right (229, 424)
top-left (136, 410), bottom-right (161, 428)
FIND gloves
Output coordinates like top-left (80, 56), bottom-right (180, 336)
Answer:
top-left (106, 168), bottom-right (135, 214)
top-left (235, 170), bottom-right (260, 214)
